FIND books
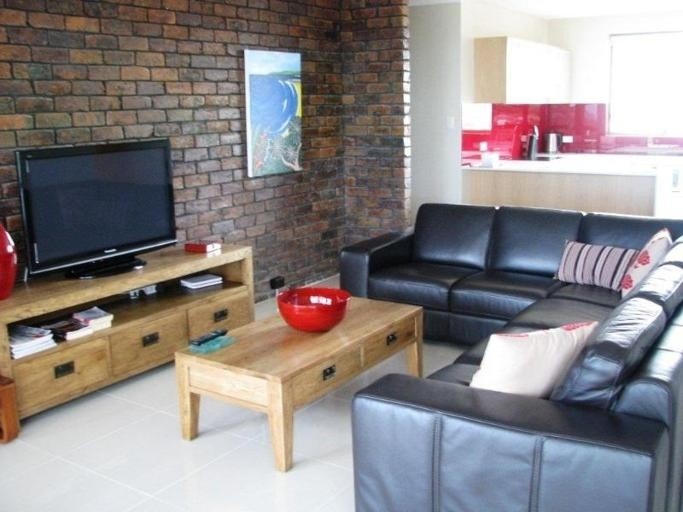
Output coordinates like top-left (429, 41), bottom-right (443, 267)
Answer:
top-left (185, 241), bottom-right (221, 253)
top-left (7, 305), bottom-right (114, 361)
top-left (180, 280), bottom-right (222, 289)
top-left (181, 273), bottom-right (222, 286)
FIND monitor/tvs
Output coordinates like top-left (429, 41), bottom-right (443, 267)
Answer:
top-left (16, 136), bottom-right (177, 280)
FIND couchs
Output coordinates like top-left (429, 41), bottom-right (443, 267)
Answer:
top-left (337, 204), bottom-right (683, 512)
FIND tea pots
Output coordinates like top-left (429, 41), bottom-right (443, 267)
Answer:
top-left (545, 132), bottom-right (562, 153)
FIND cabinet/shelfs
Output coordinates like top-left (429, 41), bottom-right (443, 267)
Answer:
top-left (2, 241), bottom-right (256, 425)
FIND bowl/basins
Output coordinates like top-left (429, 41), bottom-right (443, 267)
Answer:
top-left (277, 285), bottom-right (352, 333)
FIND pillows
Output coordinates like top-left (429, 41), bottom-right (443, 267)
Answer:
top-left (469, 320), bottom-right (600, 400)
top-left (552, 228), bottom-right (674, 301)
